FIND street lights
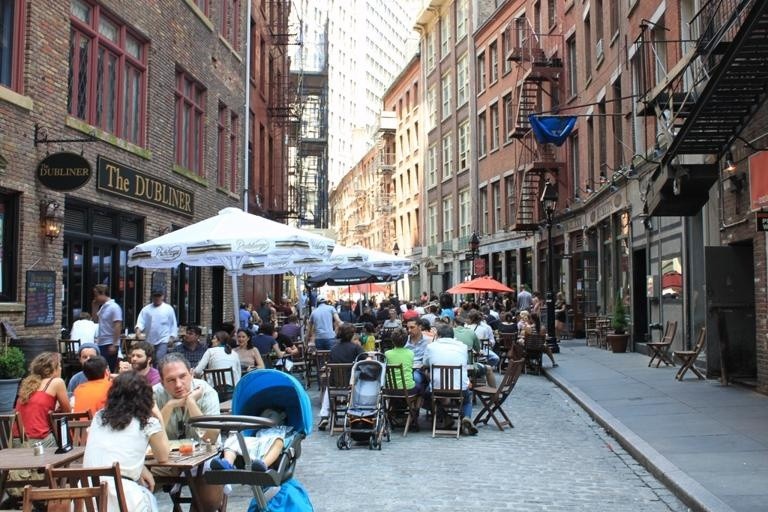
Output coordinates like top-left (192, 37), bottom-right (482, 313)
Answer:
top-left (540, 184), bottom-right (561, 354)
top-left (470, 234), bottom-right (478, 303)
top-left (394, 245), bottom-right (399, 297)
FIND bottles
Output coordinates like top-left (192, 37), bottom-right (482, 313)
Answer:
top-left (34, 443), bottom-right (40, 456)
top-left (206, 439), bottom-right (212, 452)
top-left (191, 439), bottom-right (195, 452)
top-left (38, 442), bottom-right (44, 455)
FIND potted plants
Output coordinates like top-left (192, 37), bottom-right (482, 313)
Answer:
top-left (0, 348), bottom-right (26, 411)
top-left (606, 292), bottom-right (630, 354)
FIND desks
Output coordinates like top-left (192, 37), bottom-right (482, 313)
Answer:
top-left (0, 447), bottom-right (84, 510)
top-left (119, 338), bottom-right (145, 351)
top-left (144, 443), bottom-right (219, 512)
top-left (269, 355), bottom-right (293, 373)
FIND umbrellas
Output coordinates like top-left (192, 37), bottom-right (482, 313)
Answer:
top-left (241, 247), bottom-right (367, 349)
top-left (310, 249), bottom-right (412, 335)
top-left (445, 278), bottom-right (487, 309)
top-left (341, 284), bottom-right (391, 304)
top-left (130, 205), bottom-right (337, 338)
top-left (461, 276), bottom-right (514, 309)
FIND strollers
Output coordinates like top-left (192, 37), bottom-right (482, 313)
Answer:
top-left (336, 352), bottom-right (391, 451)
top-left (185, 369), bottom-right (311, 512)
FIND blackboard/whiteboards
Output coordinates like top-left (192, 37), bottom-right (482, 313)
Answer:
top-left (26, 271), bottom-right (56, 324)
top-left (151, 271), bottom-right (167, 302)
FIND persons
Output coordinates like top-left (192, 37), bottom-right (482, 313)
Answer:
top-left (405, 317), bottom-right (433, 395)
top-left (383, 326), bottom-right (422, 417)
top-left (134, 285), bottom-right (177, 367)
top-left (238, 301), bottom-right (251, 330)
top-left (69, 313), bottom-right (98, 356)
top-left (287, 298), bottom-right (297, 324)
top-left (191, 331), bottom-right (241, 396)
top-left (479, 313), bottom-right (500, 372)
top-left (94, 284), bottom-right (123, 374)
top-left (380, 308), bottom-right (402, 336)
top-left (317, 322), bottom-right (372, 429)
top-left (449, 316), bottom-right (497, 389)
top-left (250, 322), bottom-right (286, 369)
top-left (74, 356), bottom-right (113, 423)
top-left (257, 300), bottom-right (273, 336)
top-left (517, 283), bottom-right (532, 312)
top-left (247, 302), bottom-right (262, 324)
top-left (265, 297), bottom-right (278, 329)
top-left (423, 325), bottom-right (478, 437)
top-left (173, 324), bottom-right (209, 369)
top-left (127, 342), bottom-right (162, 386)
top-left (10, 353), bottom-right (71, 448)
top-left (532, 289), bottom-right (544, 322)
top-left (221, 320), bottom-right (238, 349)
top-left (269, 324), bottom-right (298, 355)
top-left (518, 311), bottom-right (530, 334)
top-left (232, 328), bottom-right (265, 376)
top-left (329, 293), bottom-right (517, 333)
top-left (462, 308), bottom-right (490, 364)
top-left (210, 407), bottom-right (297, 472)
top-left (556, 291), bottom-right (566, 342)
top-left (518, 313), bottom-right (559, 368)
top-left (496, 314), bottom-right (518, 365)
top-left (69, 371), bottom-right (169, 512)
top-left (66, 343), bottom-right (101, 398)
top-left (275, 295), bottom-right (293, 324)
top-left (356, 323), bottom-right (376, 358)
top-left (303, 294), bottom-right (339, 390)
top-left (152, 352), bottom-right (224, 512)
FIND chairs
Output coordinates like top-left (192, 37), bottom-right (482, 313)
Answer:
top-left (326, 364), bottom-right (353, 437)
top-left (48, 409), bottom-right (93, 446)
top-left (586, 182), bottom-right (598, 190)
top-left (382, 364), bottom-right (419, 437)
top-left (675, 328), bottom-right (705, 383)
top-left (584, 318), bottom-right (599, 347)
top-left (58, 340), bottom-right (81, 369)
top-left (203, 367), bottom-right (235, 400)
top-left (1, 413), bottom-right (26, 449)
top-left (23, 482), bottom-right (108, 512)
top-left (502, 334), bottom-right (516, 350)
top-left (473, 359), bottom-right (525, 432)
top-left (375, 326), bottom-right (399, 350)
top-left (525, 334), bottom-right (545, 376)
top-left (304, 352), bottom-right (321, 391)
top-left (647, 322), bottom-right (677, 369)
top-left (431, 365), bottom-right (464, 440)
top-left (45, 462), bottom-right (128, 511)
top-left (67, 421), bottom-right (91, 446)
top-left (292, 342), bottom-right (306, 365)
top-left (479, 339), bottom-right (489, 358)
top-left (316, 352), bottom-right (332, 392)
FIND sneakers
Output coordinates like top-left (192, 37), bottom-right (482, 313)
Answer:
top-left (463, 418), bottom-right (478, 435)
top-left (442, 415), bottom-right (454, 429)
top-left (319, 417), bottom-right (328, 429)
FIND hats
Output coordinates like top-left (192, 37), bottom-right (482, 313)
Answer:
top-left (316, 295), bottom-right (327, 302)
top-left (78, 343), bottom-right (101, 354)
top-left (151, 287), bottom-right (164, 297)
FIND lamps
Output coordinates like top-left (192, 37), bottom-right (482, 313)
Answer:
top-left (721, 135), bottom-right (760, 172)
top-left (610, 172), bottom-right (629, 193)
top-left (653, 132), bottom-right (665, 160)
top-left (41, 202), bottom-right (65, 243)
top-left (627, 154), bottom-right (660, 177)
top-left (575, 194), bottom-right (579, 199)
top-left (599, 164), bottom-right (621, 178)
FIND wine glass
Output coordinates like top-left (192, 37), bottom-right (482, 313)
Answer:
top-left (194, 427), bottom-right (209, 452)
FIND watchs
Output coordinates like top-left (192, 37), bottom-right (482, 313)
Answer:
top-left (111, 343), bottom-right (118, 348)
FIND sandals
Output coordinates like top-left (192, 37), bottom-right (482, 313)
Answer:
top-left (250, 461), bottom-right (268, 474)
top-left (210, 458), bottom-right (233, 473)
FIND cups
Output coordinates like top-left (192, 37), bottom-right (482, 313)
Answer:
top-left (179, 438), bottom-right (194, 456)
